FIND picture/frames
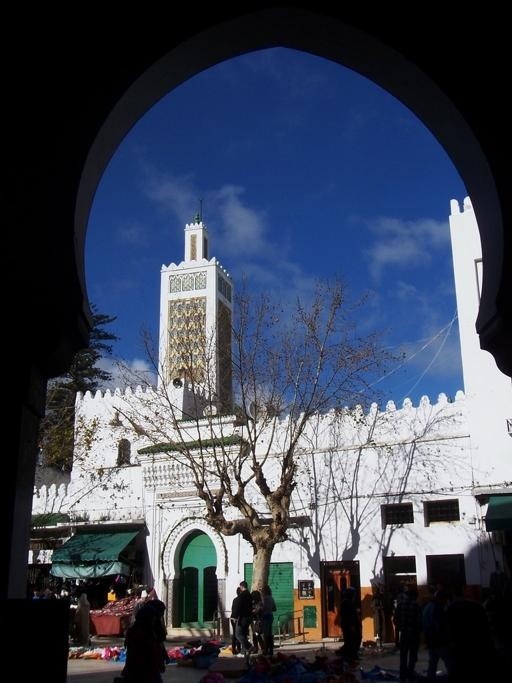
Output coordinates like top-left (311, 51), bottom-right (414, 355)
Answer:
top-left (297, 579), bottom-right (314, 599)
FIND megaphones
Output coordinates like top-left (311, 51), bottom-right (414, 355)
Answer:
top-left (173, 378), bottom-right (182, 388)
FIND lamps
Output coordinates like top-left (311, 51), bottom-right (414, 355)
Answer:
top-left (109, 409), bottom-right (123, 426)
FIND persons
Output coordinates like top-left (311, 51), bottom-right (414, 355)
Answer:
top-left (201, 652), bottom-right (359, 681)
top-left (335, 588), bottom-right (363, 662)
top-left (31, 588), bottom-right (91, 645)
top-left (230, 582), bottom-right (277, 656)
top-left (113, 587), bottom-right (171, 682)
top-left (393, 573), bottom-right (511, 682)
top-left (108, 584), bottom-right (119, 601)
